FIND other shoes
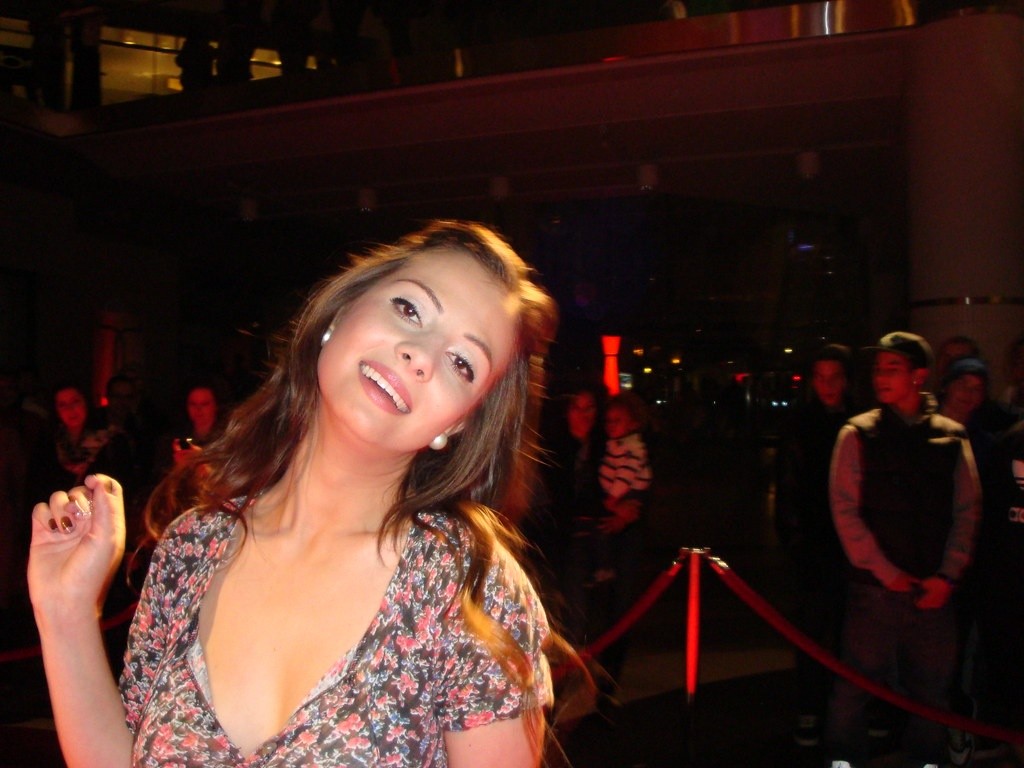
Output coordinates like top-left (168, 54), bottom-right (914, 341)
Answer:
top-left (598, 696), bottom-right (623, 726)
top-left (948, 696), bottom-right (975, 765)
top-left (868, 709), bottom-right (892, 737)
top-left (793, 711), bottom-right (820, 746)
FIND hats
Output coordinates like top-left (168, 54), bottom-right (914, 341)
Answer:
top-left (860, 331), bottom-right (936, 370)
top-left (944, 355), bottom-right (990, 389)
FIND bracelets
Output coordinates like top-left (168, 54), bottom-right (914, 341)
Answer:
top-left (937, 571), bottom-right (957, 585)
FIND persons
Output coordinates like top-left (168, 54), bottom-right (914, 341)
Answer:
top-left (87, 377), bottom-right (157, 502)
top-left (571, 402), bottom-right (652, 587)
top-left (0, 362), bottom-right (30, 696)
top-left (938, 339), bottom-right (1024, 434)
top-left (29, 221), bottom-right (555, 768)
top-left (528, 389), bottom-right (646, 732)
top-left (937, 361), bottom-right (1024, 768)
top-left (32, 380), bottom-right (143, 501)
top-left (767, 342), bottom-right (880, 745)
top-left (149, 375), bottom-right (230, 492)
top-left (825, 332), bottom-right (984, 768)
